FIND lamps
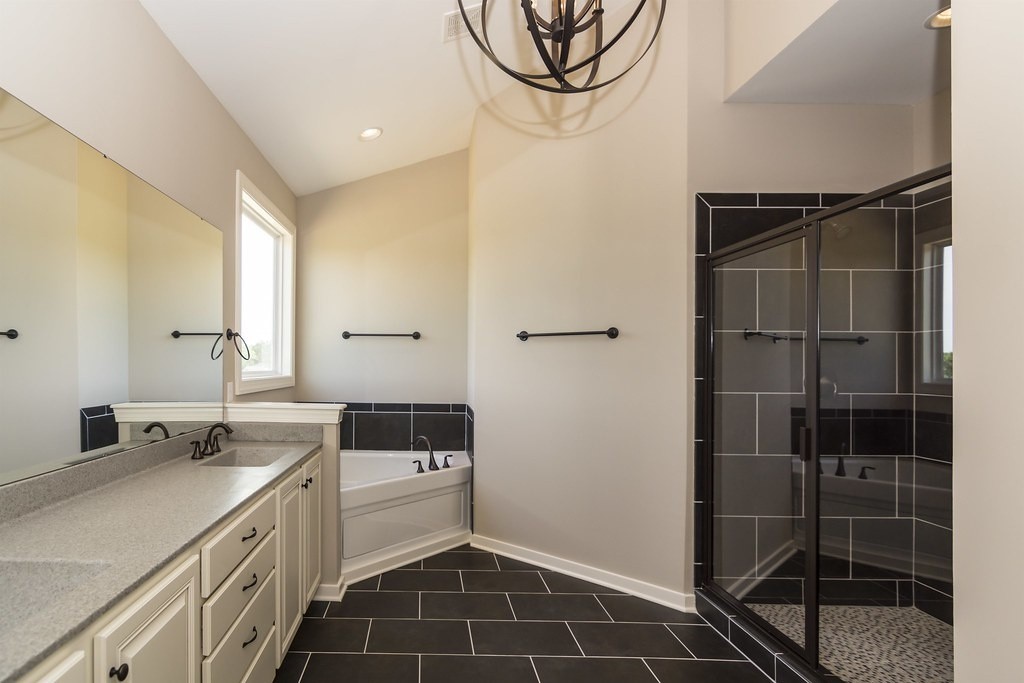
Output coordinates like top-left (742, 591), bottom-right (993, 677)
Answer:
top-left (458, 0), bottom-right (666, 94)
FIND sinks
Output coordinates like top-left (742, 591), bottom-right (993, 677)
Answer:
top-left (197, 447), bottom-right (293, 468)
top-left (65, 447), bottom-right (134, 466)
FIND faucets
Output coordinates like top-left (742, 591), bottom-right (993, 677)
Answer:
top-left (832, 441), bottom-right (848, 476)
top-left (203, 423), bottom-right (234, 455)
top-left (408, 436), bottom-right (439, 471)
top-left (143, 422), bottom-right (169, 442)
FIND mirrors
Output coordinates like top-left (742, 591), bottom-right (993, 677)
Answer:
top-left (0, 87), bottom-right (225, 487)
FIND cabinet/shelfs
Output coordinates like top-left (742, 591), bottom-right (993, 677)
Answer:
top-left (274, 452), bottom-right (322, 669)
top-left (199, 489), bottom-right (277, 683)
top-left (33, 541), bottom-right (200, 683)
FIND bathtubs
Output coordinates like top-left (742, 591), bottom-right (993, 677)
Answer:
top-left (790, 452), bottom-right (956, 581)
top-left (339, 451), bottom-right (473, 586)
